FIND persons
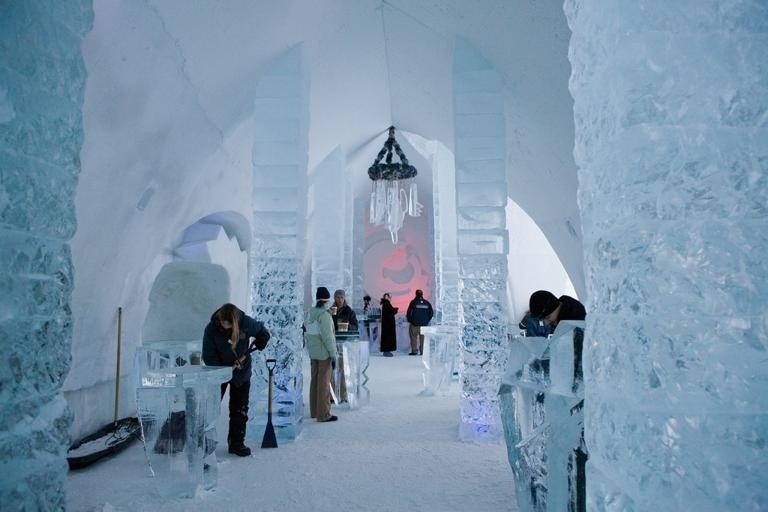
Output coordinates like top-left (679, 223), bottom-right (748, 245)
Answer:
top-left (362, 295), bottom-right (380, 344)
top-left (194, 303), bottom-right (270, 457)
top-left (407, 289), bottom-right (433, 356)
top-left (303, 287), bottom-right (338, 423)
top-left (517, 291), bottom-right (587, 512)
top-left (326, 289), bottom-right (359, 402)
top-left (379, 293), bottom-right (399, 357)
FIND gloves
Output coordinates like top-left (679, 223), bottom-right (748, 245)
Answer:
top-left (332, 357), bottom-right (337, 369)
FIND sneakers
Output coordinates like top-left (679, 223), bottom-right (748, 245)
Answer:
top-left (317, 416), bottom-right (338, 422)
top-left (228, 442), bottom-right (250, 455)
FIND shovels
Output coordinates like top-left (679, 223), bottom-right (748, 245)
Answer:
top-left (260, 359), bottom-right (278, 448)
top-left (153, 339), bottom-right (260, 455)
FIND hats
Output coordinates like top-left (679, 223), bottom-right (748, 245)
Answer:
top-left (316, 287), bottom-right (331, 302)
top-left (529, 290), bottom-right (560, 320)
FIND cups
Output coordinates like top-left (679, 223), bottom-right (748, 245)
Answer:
top-left (337, 323), bottom-right (343, 332)
top-left (343, 322), bottom-right (349, 332)
top-left (331, 307), bottom-right (337, 315)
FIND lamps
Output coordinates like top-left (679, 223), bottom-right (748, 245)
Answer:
top-left (365, 123), bottom-right (424, 248)
